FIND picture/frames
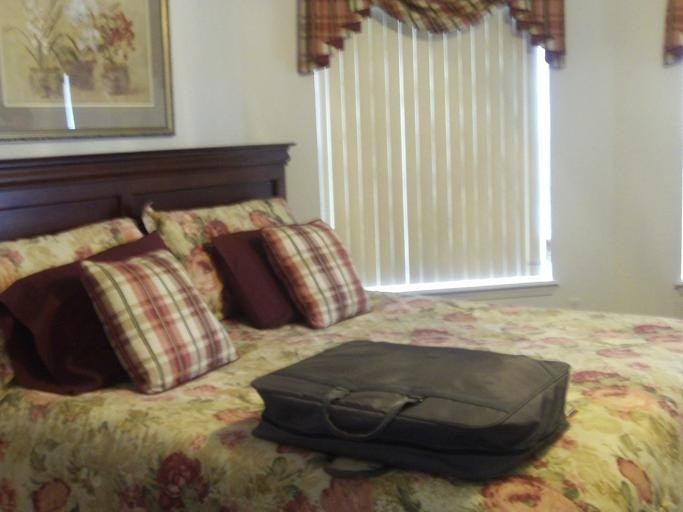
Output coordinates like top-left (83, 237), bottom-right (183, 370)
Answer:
top-left (1, 0), bottom-right (176, 139)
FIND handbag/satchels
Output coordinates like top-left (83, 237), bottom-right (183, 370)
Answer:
top-left (251, 340), bottom-right (570, 482)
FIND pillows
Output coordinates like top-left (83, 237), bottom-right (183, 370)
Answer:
top-left (0, 214), bottom-right (144, 293)
top-left (260, 218), bottom-right (372, 330)
top-left (0, 229), bottom-right (181, 394)
top-left (210, 226), bottom-right (305, 329)
top-left (78, 248), bottom-right (239, 395)
top-left (140, 193), bottom-right (297, 320)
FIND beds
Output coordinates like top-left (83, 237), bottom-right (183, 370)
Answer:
top-left (1, 141), bottom-right (683, 512)
